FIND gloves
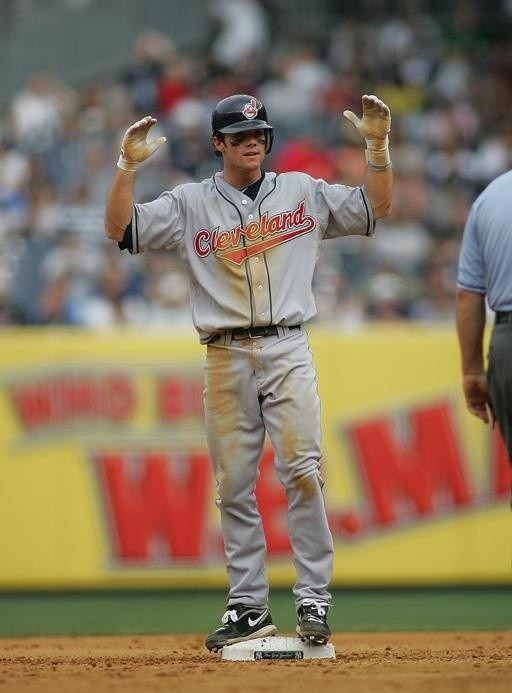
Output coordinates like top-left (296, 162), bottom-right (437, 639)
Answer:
top-left (117, 116), bottom-right (167, 171)
top-left (343, 95), bottom-right (391, 150)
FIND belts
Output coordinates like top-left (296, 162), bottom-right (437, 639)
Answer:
top-left (212, 326), bottom-right (299, 342)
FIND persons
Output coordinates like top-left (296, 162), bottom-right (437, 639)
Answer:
top-left (453, 162), bottom-right (511, 471)
top-left (0, 0), bottom-right (512, 330)
top-left (104, 82), bottom-right (397, 660)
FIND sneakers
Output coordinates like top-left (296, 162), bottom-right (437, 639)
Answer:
top-left (296, 600), bottom-right (331, 644)
top-left (206, 603), bottom-right (277, 653)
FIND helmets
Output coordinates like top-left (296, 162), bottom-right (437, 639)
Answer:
top-left (213, 94), bottom-right (274, 138)
